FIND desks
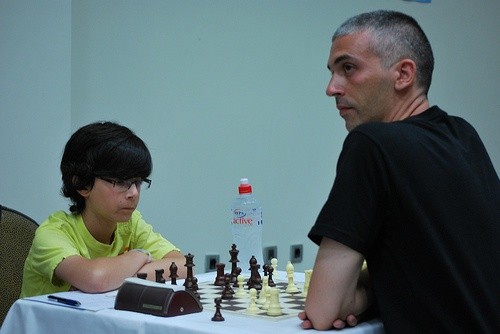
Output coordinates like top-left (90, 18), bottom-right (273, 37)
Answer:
top-left (0, 270), bottom-right (383, 334)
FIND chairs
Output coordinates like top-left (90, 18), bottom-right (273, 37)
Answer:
top-left (0, 204), bottom-right (40, 328)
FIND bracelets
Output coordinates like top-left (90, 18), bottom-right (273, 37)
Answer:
top-left (127, 249), bottom-right (152, 264)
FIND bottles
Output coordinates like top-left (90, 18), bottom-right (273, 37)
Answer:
top-left (229, 177), bottom-right (265, 276)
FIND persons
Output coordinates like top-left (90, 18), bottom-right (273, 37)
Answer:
top-left (20, 122), bottom-right (194, 299)
top-left (298, 10), bottom-right (500, 334)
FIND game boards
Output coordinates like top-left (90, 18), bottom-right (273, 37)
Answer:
top-left (178, 274), bottom-right (306, 321)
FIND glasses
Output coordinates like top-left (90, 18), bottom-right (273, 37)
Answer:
top-left (95, 175), bottom-right (152, 193)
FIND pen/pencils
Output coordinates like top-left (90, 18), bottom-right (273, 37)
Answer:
top-left (47, 295), bottom-right (81, 307)
